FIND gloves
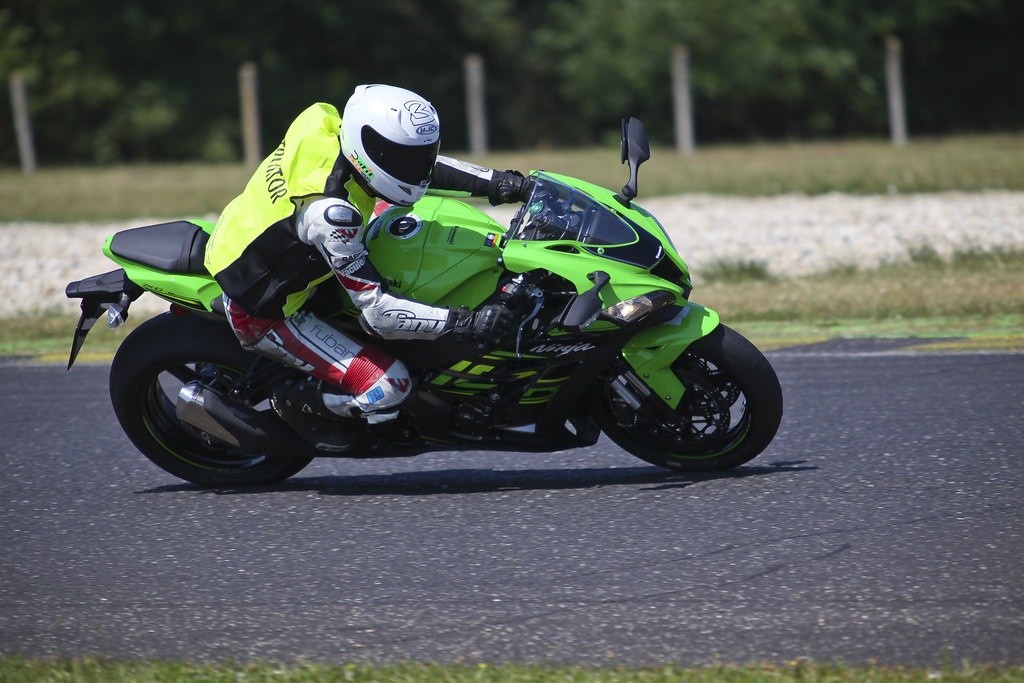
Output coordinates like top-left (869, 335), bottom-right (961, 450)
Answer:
top-left (488, 168), bottom-right (558, 206)
top-left (451, 305), bottom-right (516, 346)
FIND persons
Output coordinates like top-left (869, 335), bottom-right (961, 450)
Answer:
top-left (202, 80), bottom-right (527, 456)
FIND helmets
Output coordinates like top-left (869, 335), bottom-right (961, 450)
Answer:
top-left (340, 84), bottom-right (441, 208)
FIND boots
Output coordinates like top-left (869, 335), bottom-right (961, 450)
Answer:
top-left (269, 374), bottom-right (358, 452)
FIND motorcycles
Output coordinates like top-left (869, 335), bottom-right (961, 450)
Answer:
top-left (63, 115), bottom-right (783, 494)
